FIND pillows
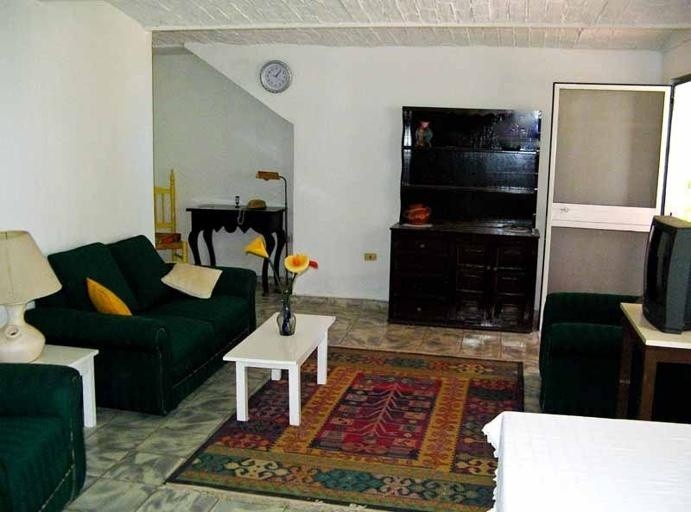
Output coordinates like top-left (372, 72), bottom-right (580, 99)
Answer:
top-left (108, 234), bottom-right (170, 310)
top-left (161, 262), bottom-right (223, 299)
top-left (86, 277), bottom-right (133, 315)
top-left (47, 242), bottom-right (137, 314)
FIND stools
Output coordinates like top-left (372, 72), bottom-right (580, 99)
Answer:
top-left (539, 292), bottom-right (641, 418)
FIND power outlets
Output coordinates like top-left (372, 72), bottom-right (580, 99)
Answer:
top-left (364, 253), bottom-right (376, 261)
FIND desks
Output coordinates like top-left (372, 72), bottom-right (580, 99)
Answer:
top-left (616, 302), bottom-right (691, 420)
top-left (481, 410), bottom-right (691, 512)
top-left (185, 203), bottom-right (288, 298)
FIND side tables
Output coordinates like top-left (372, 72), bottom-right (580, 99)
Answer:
top-left (32, 343), bottom-right (100, 427)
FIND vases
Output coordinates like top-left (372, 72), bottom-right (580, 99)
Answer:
top-left (276, 290), bottom-right (295, 335)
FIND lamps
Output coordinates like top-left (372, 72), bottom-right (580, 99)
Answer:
top-left (255, 170), bottom-right (288, 293)
top-left (0, 229), bottom-right (62, 363)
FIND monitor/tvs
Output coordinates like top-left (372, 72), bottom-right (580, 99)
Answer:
top-left (643, 216), bottom-right (691, 334)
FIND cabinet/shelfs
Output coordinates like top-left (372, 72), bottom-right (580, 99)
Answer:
top-left (400, 106), bottom-right (541, 194)
top-left (388, 220), bottom-right (540, 333)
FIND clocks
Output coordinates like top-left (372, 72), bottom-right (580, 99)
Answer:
top-left (260, 60), bottom-right (292, 93)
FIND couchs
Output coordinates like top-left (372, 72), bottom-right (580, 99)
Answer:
top-left (24, 264), bottom-right (256, 416)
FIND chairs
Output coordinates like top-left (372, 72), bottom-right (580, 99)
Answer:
top-left (0, 364), bottom-right (86, 512)
top-left (154, 169), bottom-right (188, 265)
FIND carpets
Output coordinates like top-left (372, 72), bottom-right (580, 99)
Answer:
top-left (162, 345), bottom-right (524, 512)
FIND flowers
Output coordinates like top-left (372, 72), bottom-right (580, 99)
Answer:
top-left (243, 237), bottom-right (318, 290)
